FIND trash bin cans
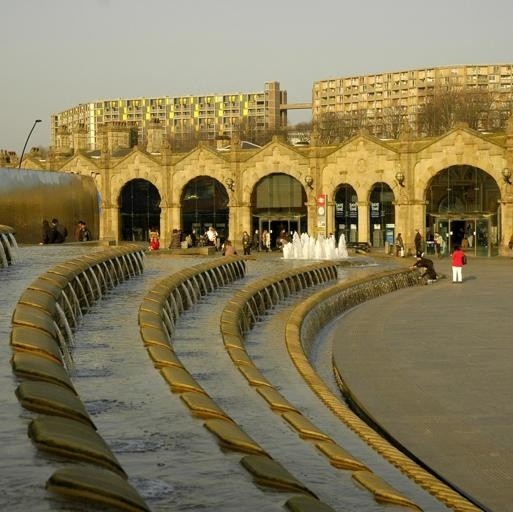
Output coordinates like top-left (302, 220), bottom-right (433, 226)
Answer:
top-left (426, 241), bottom-right (435, 255)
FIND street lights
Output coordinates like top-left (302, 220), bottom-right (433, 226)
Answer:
top-left (297, 131), bottom-right (304, 141)
top-left (17, 118), bottom-right (42, 168)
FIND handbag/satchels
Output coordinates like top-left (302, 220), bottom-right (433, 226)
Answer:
top-left (463, 256), bottom-right (467, 264)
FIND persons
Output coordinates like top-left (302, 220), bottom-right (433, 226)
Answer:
top-left (408, 255), bottom-right (436, 281)
top-left (148, 226), bottom-right (294, 255)
top-left (413, 228), bottom-right (422, 260)
top-left (394, 233), bottom-right (403, 256)
top-left (450, 244), bottom-right (464, 283)
top-left (433, 231), bottom-right (442, 259)
top-left (37, 218), bottom-right (92, 245)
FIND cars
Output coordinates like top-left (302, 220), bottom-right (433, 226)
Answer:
top-left (293, 141), bottom-right (309, 147)
